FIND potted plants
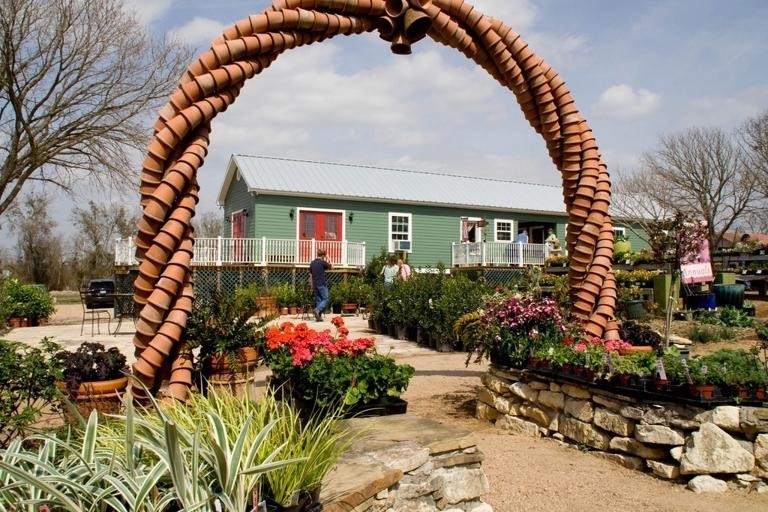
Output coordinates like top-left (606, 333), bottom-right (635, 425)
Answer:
top-left (1, 377), bottom-right (359, 512)
top-left (193, 269), bottom-right (455, 354)
top-left (456, 256), bottom-right (768, 404)
top-left (2, 326), bottom-right (414, 435)
top-left (2, 272), bottom-right (60, 326)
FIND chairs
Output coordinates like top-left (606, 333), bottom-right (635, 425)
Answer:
top-left (77, 269), bottom-right (138, 337)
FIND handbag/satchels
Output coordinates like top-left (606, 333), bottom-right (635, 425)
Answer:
top-left (379, 274), bottom-right (385, 280)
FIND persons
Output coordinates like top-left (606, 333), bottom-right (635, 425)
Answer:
top-left (544, 228), bottom-right (556, 244)
top-left (516, 229), bottom-right (528, 243)
top-left (309, 249), bottom-right (333, 322)
top-left (736, 234), bottom-right (751, 251)
top-left (380, 255), bottom-right (410, 285)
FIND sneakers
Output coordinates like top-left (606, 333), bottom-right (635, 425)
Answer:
top-left (313, 308), bottom-right (323, 321)
